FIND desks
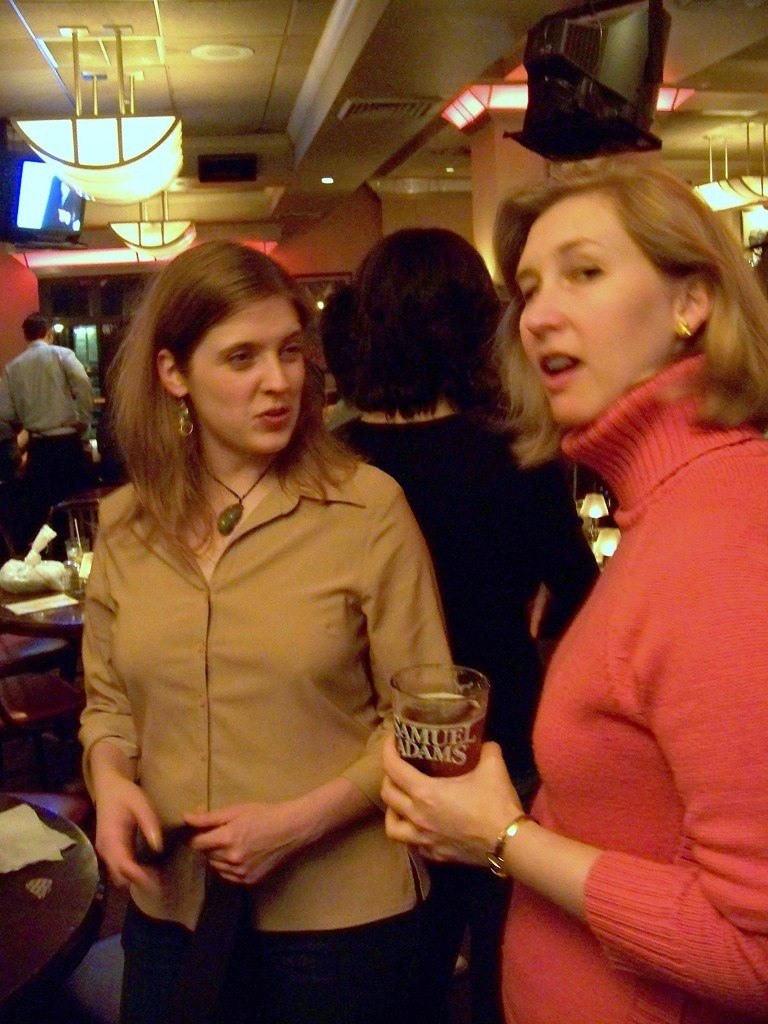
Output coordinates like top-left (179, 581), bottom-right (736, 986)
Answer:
top-left (0, 796), bottom-right (109, 1024)
top-left (0, 590), bottom-right (86, 637)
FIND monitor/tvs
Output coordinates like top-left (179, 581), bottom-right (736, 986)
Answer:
top-left (521, 0), bottom-right (670, 137)
top-left (0, 152), bottom-right (86, 242)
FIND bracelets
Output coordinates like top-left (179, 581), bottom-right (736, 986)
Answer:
top-left (484, 814), bottom-right (542, 879)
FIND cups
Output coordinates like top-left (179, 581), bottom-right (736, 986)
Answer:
top-left (389, 665), bottom-right (491, 780)
top-left (65, 538), bottom-right (90, 591)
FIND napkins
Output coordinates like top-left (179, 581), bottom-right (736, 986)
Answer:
top-left (4, 593), bottom-right (79, 616)
top-left (0, 803), bottom-right (77, 873)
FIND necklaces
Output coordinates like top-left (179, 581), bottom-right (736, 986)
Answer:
top-left (198, 445), bottom-right (277, 536)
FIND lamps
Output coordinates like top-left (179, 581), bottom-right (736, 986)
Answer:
top-left (577, 492), bottom-right (622, 566)
top-left (727, 121), bottom-right (768, 205)
top-left (108, 191), bottom-right (196, 257)
top-left (693, 134), bottom-right (752, 213)
top-left (10, 26), bottom-right (184, 206)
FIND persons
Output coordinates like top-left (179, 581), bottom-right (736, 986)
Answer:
top-left (380, 149), bottom-right (768, 1024)
top-left (79, 239), bottom-right (463, 1024)
top-left (0, 225), bottom-right (601, 1024)
